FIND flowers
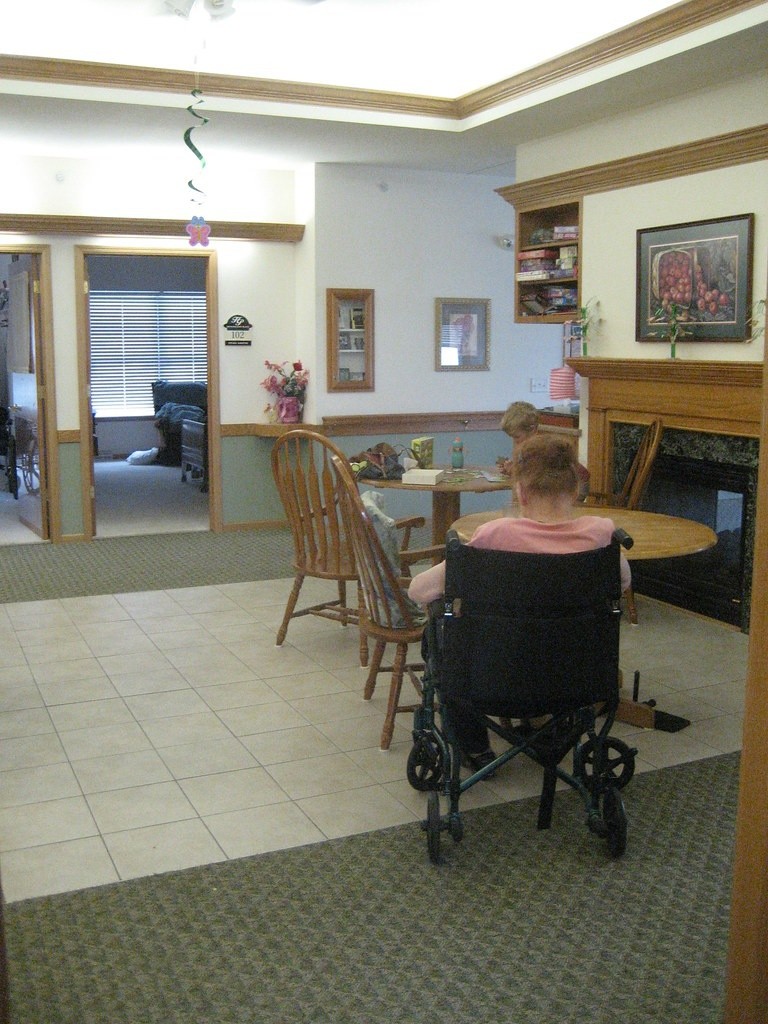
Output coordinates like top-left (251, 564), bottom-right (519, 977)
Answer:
top-left (260, 359), bottom-right (310, 399)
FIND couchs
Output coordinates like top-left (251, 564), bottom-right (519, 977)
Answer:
top-left (152, 380), bottom-right (207, 467)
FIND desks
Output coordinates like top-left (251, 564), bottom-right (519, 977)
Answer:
top-left (449, 506), bottom-right (718, 732)
top-left (358, 462), bottom-right (513, 567)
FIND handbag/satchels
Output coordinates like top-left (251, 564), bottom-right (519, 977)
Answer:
top-left (359, 442), bottom-right (427, 479)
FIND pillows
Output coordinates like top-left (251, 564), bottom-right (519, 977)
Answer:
top-left (353, 490), bottom-right (427, 629)
top-left (125, 446), bottom-right (159, 465)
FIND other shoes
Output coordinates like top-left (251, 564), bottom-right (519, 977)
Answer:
top-left (459, 751), bottom-right (496, 780)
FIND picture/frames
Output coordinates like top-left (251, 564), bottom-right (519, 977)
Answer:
top-left (636, 212), bottom-right (756, 344)
top-left (435, 298), bottom-right (490, 372)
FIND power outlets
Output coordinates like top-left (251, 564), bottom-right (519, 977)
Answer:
top-left (530, 377), bottom-right (548, 393)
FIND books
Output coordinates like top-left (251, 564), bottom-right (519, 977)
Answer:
top-left (515, 249), bottom-right (577, 281)
top-left (552, 225), bottom-right (579, 240)
top-left (550, 287), bottom-right (577, 307)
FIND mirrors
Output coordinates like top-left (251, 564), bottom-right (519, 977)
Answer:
top-left (326, 287), bottom-right (375, 393)
top-left (574, 416), bottom-right (663, 627)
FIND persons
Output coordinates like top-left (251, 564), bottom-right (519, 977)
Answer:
top-left (495, 400), bottom-right (591, 505)
top-left (407, 433), bottom-right (632, 780)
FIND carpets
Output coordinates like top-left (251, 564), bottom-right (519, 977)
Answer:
top-left (0, 525), bottom-right (434, 603)
top-left (2, 751), bottom-right (742, 1024)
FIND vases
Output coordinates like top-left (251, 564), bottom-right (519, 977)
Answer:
top-left (278, 396), bottom-right (299, 424)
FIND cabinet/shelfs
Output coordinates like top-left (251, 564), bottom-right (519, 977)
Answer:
top-left (338, 328), bottom-right (365, 381)
top-left (514, 196), bottom-right (583, 325)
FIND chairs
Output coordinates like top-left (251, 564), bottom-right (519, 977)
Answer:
top-left (271, 430), bottom-right (425, 648)
top-left (0, 407), bottom-right (18, 500)
top-left (181, 419), bottom-right (209, 493)
top-left (331, 455), bottom-right (513, 751)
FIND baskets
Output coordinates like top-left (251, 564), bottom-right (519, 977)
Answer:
top-left (651, 245), bottom-right (711, 309)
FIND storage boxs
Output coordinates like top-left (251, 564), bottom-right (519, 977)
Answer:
top-left (402, 469), bottom-right (445, 486)
top-left (411, 436), bottom-right (434, 468)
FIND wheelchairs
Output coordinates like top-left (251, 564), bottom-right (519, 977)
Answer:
top-left (407, 529), bottom-right (639, 862)
top-left (17, 416), bottom-right (41, 494)
top-left (0, 406), bottom-right (16, 496)
